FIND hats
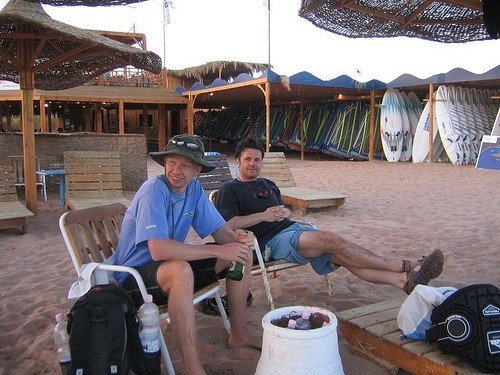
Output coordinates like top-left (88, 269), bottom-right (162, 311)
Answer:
top-left (149, 133), bottom-right (216, 173)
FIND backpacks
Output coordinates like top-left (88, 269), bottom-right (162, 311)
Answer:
top-left (57, 284), bottom-right (162, 375)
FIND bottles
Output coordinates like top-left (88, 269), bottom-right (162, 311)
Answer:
top-left (136, 294), bottom-right (161, 353)
top-left (286, 310), bottom-right (312, 330)
top-left (53, 313), bottom-right (71, 362)
top-left (224, 229), bottom-right (249, 281)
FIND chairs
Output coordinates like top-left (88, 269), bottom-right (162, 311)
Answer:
top-left (0, 151), bottom-right (346, 375)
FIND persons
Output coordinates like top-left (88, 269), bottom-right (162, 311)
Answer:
top-left (113, 134), bottom-right (263, 375)
top-left (216, 139), bottom-right (444, 295)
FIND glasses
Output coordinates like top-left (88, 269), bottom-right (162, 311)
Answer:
top-left (170, 139), bottom-right (204, 158)
top-left (256, 189), bottom-right (272, 199)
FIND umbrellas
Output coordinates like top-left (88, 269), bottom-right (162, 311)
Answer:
top-left (0, 0), bottom-right (162, 220)
top-left (297, 0), bottom-right (500, 43)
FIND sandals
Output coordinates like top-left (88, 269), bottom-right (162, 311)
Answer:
top-left (401, 248), bottom-right (444, 295)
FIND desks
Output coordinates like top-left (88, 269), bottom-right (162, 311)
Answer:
top-left (335, 297), bottom-right (487, 375)
top-left (36, 170), bottom-right (66, 207)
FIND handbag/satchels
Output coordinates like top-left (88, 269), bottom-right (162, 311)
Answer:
top-left (396, 283), bottom-right (458, 341)
top-left (425, 282), bottom-right (500, 375)
